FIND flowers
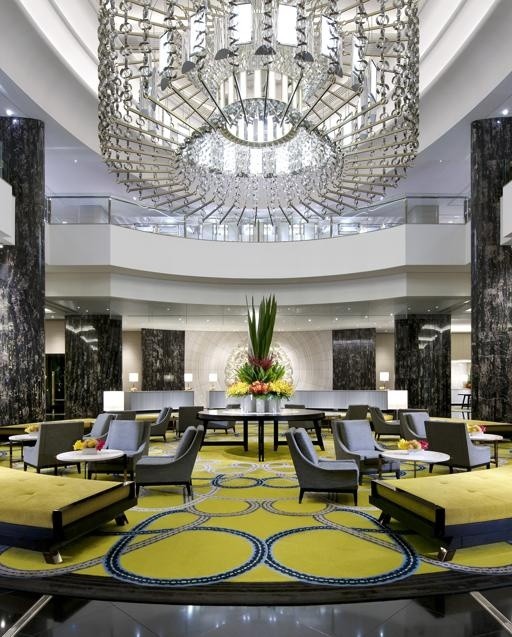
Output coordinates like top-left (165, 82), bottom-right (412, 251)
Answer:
top-left (224, 345), bottom-right (295, 401)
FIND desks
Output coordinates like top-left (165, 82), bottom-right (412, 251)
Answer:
top-left (101, 389), bottom-right (195, 413)
top-left (457, 393), bottom-right (471, 409)
top-left (207, 389), bottom-right (410, 420)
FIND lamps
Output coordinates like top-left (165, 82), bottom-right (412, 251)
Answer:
top-left (378, 372), bottom-right (389, 390)
top-left (94, 0), bottom-right (425, 234)
top-left (208, 372), bottom-right (218, 391)
top-left (183, 372), bottom-right (192, 391)
top-left (129, 372), bottom-right (140, 392)
